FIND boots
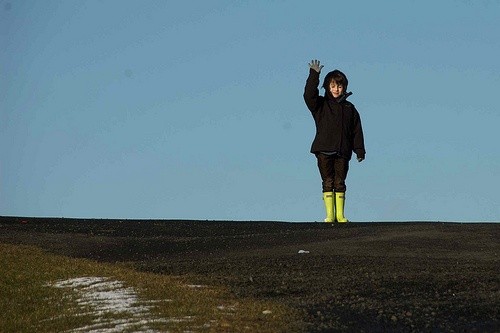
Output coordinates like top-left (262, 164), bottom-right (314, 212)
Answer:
top-left (323, 191), bottom-right (335, 222)
top-left (333, 192), bottom-right (347, 222)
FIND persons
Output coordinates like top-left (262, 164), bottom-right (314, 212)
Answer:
top-left (304, 58), bottom-right (365, 223)
